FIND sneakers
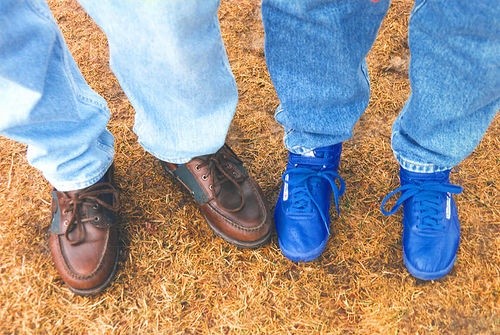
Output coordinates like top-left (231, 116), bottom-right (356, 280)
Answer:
top-left (381, 163), bottom-right (463, 281)
top-left (274, 142), bottom-right (345, 262)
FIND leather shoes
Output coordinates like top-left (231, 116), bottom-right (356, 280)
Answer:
top-left (161, 142), bottom-right (273, 249)
top-left (48, 164), bottom-right (120, 296)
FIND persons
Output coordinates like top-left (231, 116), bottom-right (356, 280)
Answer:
top-left (0, 0), bottom-right (275, 296)
top-left (261, 0), bottom-right (500, 282)
